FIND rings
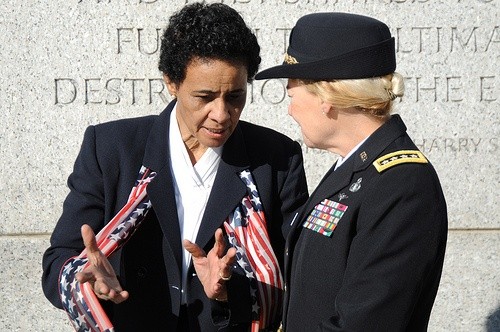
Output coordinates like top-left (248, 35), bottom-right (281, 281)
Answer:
top-left (219, 273), bottom-right (232, 280)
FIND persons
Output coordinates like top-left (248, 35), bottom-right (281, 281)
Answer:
top-left (42, 4), bottom-right (310, 332)
top-left (255, 12), bottom-right (448, 332)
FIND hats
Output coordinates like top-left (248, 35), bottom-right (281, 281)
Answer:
top-left (254, 12), bottom-right (396, 81)
